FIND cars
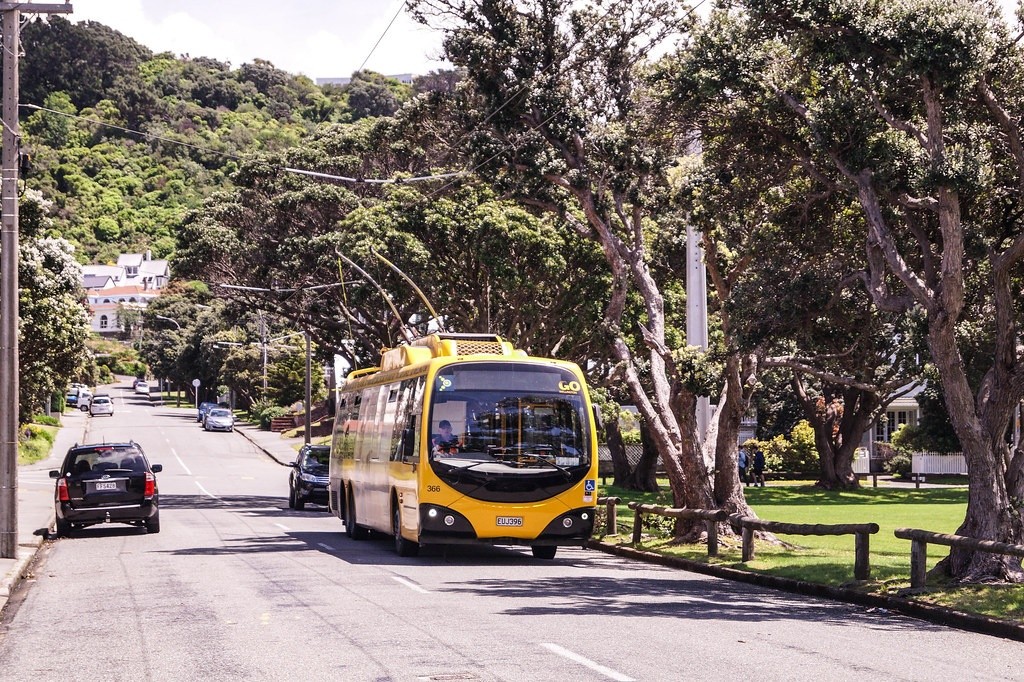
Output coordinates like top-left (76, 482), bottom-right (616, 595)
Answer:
top-left (133, 379), bottom-right (145, 388)
top-left (197, 402), bottom-right (219, 428)
top-left (135, 382), bottom-right (149, 395)
top-left (205, 409), bottom-right (237, 432)
top-left (88, 394), bottom-right (114, 417)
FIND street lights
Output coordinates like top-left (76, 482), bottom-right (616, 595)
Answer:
top-left (157, 315), bottom-right (180, 408)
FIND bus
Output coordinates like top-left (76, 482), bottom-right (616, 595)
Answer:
top-left (329, 332), bottom-right (599, 559)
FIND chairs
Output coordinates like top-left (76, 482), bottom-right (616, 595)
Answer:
top-left (120, 457), bottom-right (135, 468)
top-left (76, 460), bottom-right (91, 472)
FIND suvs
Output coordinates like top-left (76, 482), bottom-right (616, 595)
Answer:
top-left (289, 443), bottom-right (332, 510)
top-left (49, 440), bottom-right (162, 533)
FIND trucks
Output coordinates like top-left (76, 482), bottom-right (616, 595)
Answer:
top-left (66, 387), bottom-right (93, 412)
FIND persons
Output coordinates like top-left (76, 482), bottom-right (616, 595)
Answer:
top-left (750, 447), bottom-right (765, 487)
top-left (738, 445), bottom-right (749, 487)
top-left (432, 420), bottom-right (463, 455)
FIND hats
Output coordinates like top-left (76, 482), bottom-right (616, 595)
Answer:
top-left (438, 420), bottom-right (450, 428)
